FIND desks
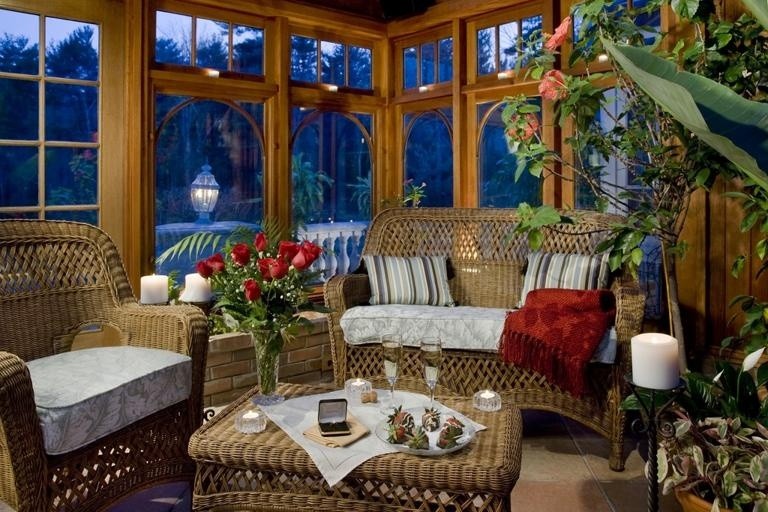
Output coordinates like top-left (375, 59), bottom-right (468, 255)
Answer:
top-left (188, 383), bottom-right (527, 512)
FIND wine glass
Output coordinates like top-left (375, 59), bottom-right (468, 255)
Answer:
top-left (381, 335), bottom-right (404, 411)
top-left (418, 336), bottom-right (443, 408)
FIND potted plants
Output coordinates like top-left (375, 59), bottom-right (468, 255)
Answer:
top-left (659, 411), bottom-right (768, 511)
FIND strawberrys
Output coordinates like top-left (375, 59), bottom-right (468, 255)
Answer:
top-left (385, 404), bottom-right (465, 449)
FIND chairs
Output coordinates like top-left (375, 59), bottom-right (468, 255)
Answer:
top-left (322, 206), bottom-right (631, 471)
top-left (0, 219), bottom-right (211, 512)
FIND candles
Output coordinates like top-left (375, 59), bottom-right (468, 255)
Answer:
top-left (632, 331), bottom-right (682, 391)
top-left (179, 273), bottom-right (214, 306)
top-left (139, 273), bottom-right (169, 305)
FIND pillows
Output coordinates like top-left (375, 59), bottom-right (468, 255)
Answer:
top-left (517, 249), bottom-right (610, 310)
top-left (361, 256), bottom-right (459, 307)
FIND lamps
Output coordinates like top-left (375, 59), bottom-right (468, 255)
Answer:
top-left (187, 163), bottom-right (221, 225)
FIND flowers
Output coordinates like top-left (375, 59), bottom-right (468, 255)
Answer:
top-left (198, 217), bottom-right (325, 388)
top-left (501, 0), bottom-right (767, 247)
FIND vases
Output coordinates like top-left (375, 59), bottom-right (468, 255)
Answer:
top-left (247, 326), bottom-right (289, 406)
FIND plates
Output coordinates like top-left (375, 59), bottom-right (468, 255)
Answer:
top-left (374, 407), bottom-right (476, 457)
